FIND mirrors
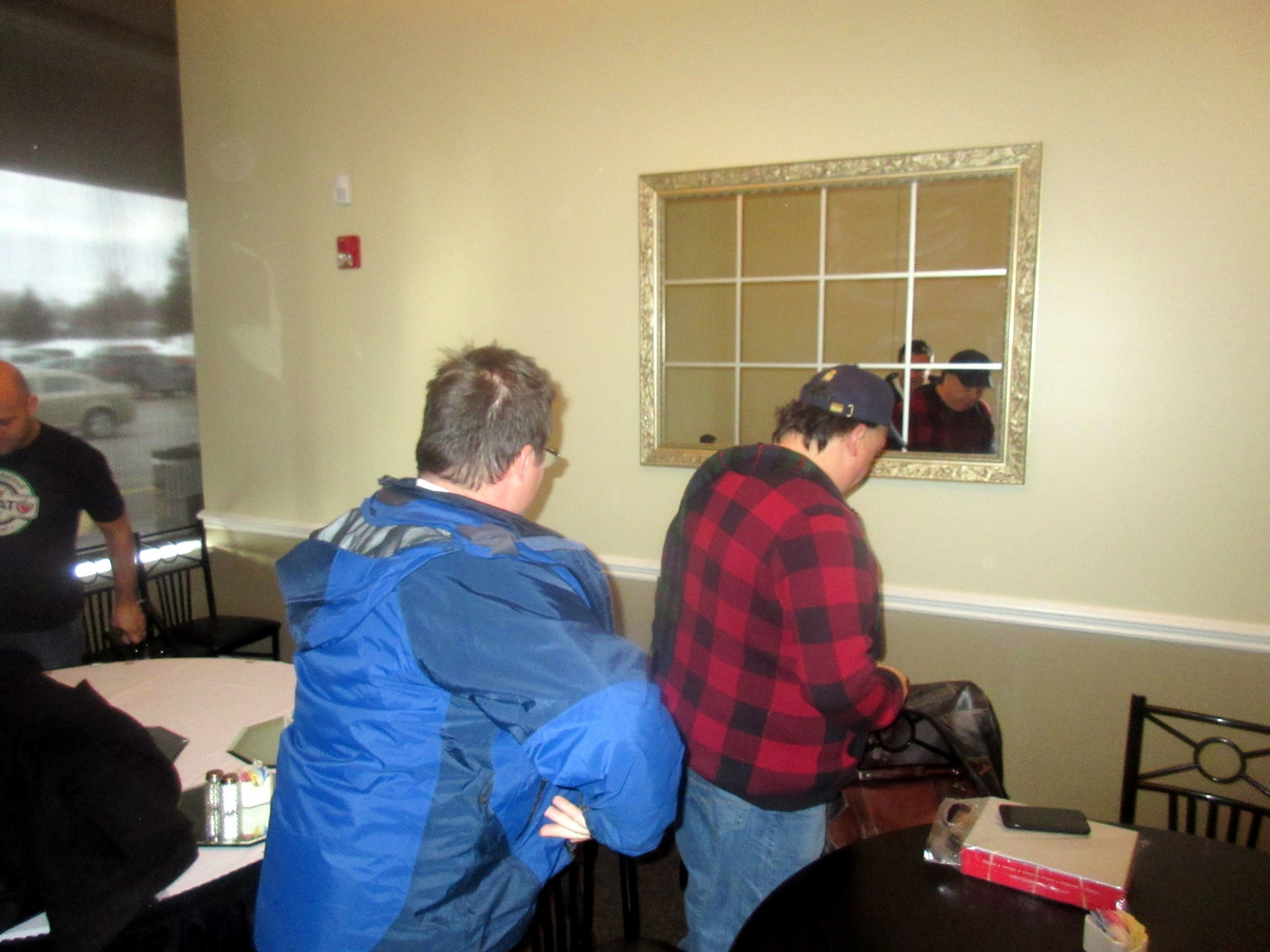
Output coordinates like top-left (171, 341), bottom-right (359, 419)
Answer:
top-left (638, 141), bottom-right (1043, 486)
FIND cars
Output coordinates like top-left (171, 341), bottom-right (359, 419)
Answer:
top-left (0, 339), bottom-right (197, 400)
top-left (23, 366), bottom-right (140, 440)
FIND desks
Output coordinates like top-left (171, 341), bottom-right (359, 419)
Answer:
top-left (729, 820), bottom-right (1270, 951)
top-left (1, 657), bottom-right (296, 952)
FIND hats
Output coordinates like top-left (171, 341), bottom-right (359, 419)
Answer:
top-left (949, 350), bottom-right (994, 388)
top-left (799, 365), bottom-right (907, 450)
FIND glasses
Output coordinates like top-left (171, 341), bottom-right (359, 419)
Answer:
top-left (541, 445), bottom-right (561, 469)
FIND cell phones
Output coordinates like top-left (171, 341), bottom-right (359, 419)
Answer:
top-left (1000, 805), bottom-right (1091, 834)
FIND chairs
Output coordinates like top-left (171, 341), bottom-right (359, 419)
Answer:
top-left (826, 680), bottom-right (1008, 851)
top-left (511, 839), bottom-right (686, 952)
top-left (1120, 694), bottom-right (1270, 849)
top-left (76, 520), bottom-right (281, 665)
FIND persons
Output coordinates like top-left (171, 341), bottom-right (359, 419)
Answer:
top-left (0, 360), bottom-right (148, 671)
top-left (883, 339), bottom-right (997, 454)
top-left (251, 347), bottom-right (685, 952)
top-left (647, 365), bottom-right (908, 952)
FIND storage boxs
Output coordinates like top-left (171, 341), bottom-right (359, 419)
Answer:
top-left (961, 796), bottom-right (1140, 911)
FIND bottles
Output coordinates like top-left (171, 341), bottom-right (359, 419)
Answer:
top-left (219, 773), bottom-right (239, 815)
top-left (203, 810), bottom-right (220, 844)
top-left (203, 768), bottom-right (223, 810)
top-left (221, 812), bottom-right (238, 844)
